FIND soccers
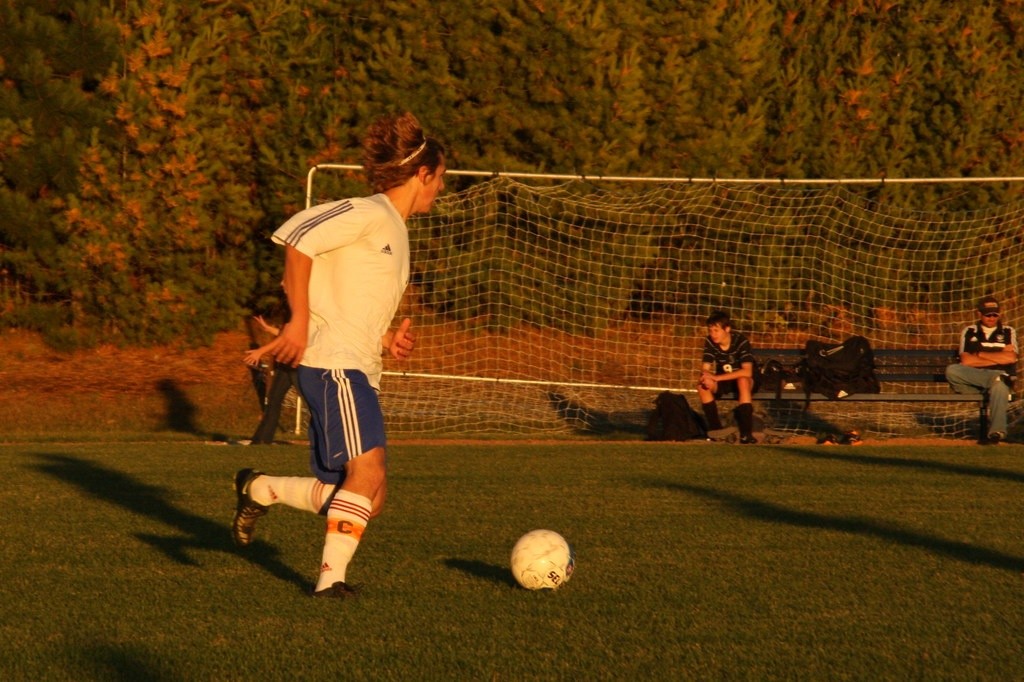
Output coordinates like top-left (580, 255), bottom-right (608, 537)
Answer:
top-left (510, 528), bottom-right (574, 594)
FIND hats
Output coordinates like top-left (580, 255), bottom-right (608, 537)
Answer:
top-left (978, 297), bottom-right (1001, 316)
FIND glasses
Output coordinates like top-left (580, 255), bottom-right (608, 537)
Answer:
top-left (984, 314), bottom-right (998, 318)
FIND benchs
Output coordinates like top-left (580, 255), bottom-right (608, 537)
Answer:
top-left (718, 348), bottom-right (1014, 444)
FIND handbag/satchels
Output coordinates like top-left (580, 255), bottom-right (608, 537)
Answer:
top-left (804, 335), bottom-right (881, 401)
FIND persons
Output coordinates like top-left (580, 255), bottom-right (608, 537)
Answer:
top-left (697, 311), bottom-right (761, 444)
top-left (945, 297), bottom-right (1019, 441)
top-left (243, 285), bottom-right (293, 444)
top-left (231, 108), bottom-right (446, 599)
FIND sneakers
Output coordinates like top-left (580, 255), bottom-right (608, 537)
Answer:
top-left (229, 467), bottom-right (270, 550)
top-left (312, 581), bottom-right (365, 600)
top-left (740, 431), bottom-right (758, 444)
top-left (990, 431), bottom-right (1006, 441)
